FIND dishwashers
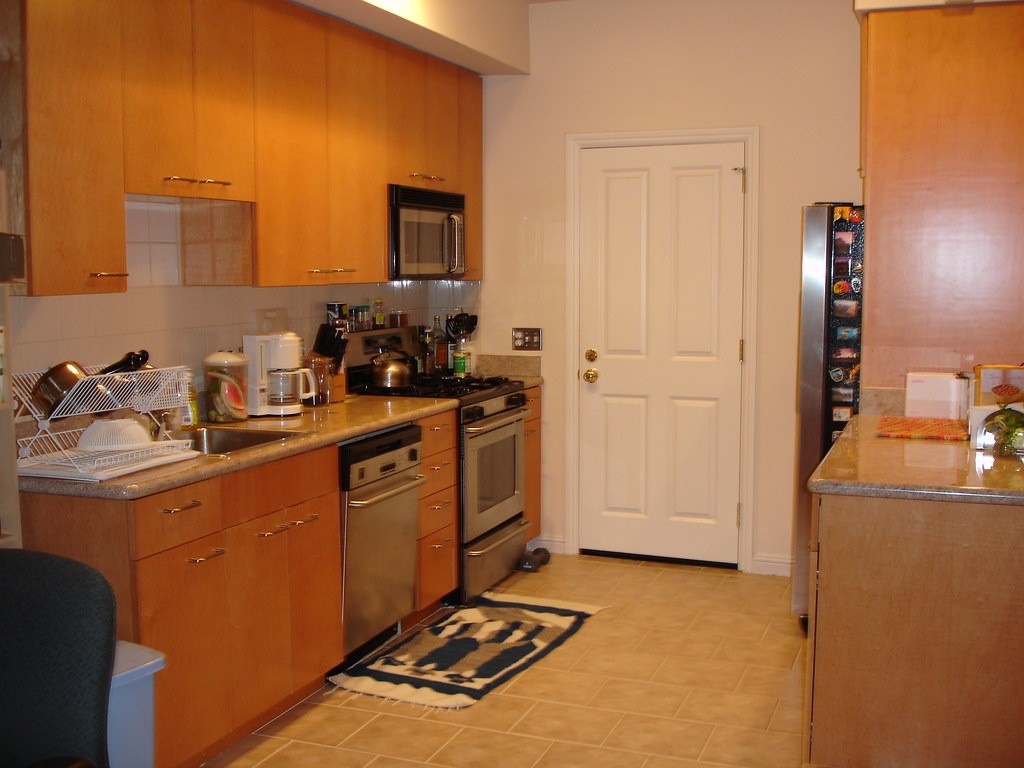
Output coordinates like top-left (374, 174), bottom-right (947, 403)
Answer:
top-left (338, 425), bottom-right (429, 656)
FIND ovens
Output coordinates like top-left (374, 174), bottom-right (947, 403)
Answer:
top-left (456, 390), bottom-right (533, 603)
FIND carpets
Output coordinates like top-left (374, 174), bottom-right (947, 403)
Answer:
top-left (320, 591), bottom-right (613, 721)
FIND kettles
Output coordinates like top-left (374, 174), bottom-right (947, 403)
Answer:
top-left (368, 345), bottom-right (417, 388)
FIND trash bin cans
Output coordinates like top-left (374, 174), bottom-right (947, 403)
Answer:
top-left (107, 639), bottom-right (166, 768)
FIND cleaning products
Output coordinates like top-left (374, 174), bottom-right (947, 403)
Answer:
top-left (165, 367), bottom-right (199, 432)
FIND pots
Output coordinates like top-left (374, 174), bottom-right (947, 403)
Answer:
top-left (31, 350), bottom-right (162, 417)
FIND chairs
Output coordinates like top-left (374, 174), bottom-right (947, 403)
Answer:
top-left (0, 548), bottom-right (119, 768)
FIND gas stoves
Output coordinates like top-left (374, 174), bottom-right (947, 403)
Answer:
top-left (346, 355), bottom-right (525, 406)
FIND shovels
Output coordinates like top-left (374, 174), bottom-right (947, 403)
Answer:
top-left (446, 312), bottom-right (477, 342)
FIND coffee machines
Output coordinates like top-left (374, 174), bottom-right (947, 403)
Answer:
top-left (243, 332), bottom-right (317, 417)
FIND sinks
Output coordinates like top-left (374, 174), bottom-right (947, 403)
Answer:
top-left (164, 425), bottom-right (301, 455)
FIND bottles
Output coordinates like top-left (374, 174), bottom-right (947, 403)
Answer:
top-left (465, 340), bottom-right (477, 375)
top-left (424, 313), bottom-right (456, 376)
top-left (174, 367), bottom-right (200, 430)
top-left (302, 356), bottom-right (329, 406)
top-left (453, 336), bottom-right (472, 377)
top-left (202, 350), bottom-right (249, 422)
top-left (373, 299), bottom-right (386, 329)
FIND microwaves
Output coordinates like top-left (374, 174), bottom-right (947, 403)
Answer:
top-left (388, 183), bottom-right (466, 280)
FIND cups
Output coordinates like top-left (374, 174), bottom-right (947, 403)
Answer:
top-left (390, 308), bottom-right (408, 327)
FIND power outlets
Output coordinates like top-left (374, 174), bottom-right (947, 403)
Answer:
top-left (512, 327), bottom-right (543, 351)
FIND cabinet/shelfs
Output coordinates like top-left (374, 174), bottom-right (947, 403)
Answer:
top-left (803, 0), bottom-right (1024, 768)
top-left (179, 0), bottom-right (388, 288)
top-left (221, 443), bottom-right (346, 744)
top-left (121, 0), bottom-right (255, 202)
top-left (389, 43), bottom-right (483, 284)
top-left (524, 385), bottom-right (542, 555)
top-left (0, 0), bottom-right (129, 298)
top-left (414, 409), bottom-right (459, 629)
top-left (19, 477), bottom-right (240, 768)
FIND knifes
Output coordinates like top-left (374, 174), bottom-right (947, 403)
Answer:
top-left (312, 323), bottom-right (348, 373)
